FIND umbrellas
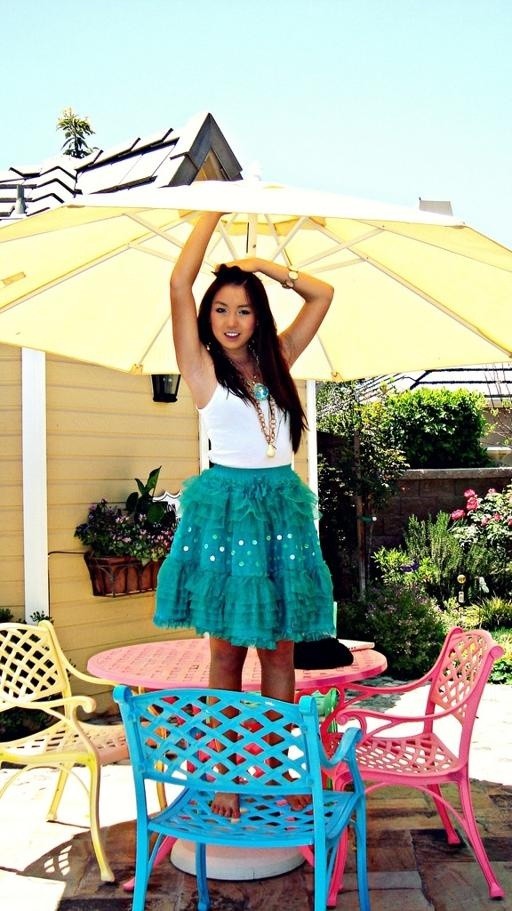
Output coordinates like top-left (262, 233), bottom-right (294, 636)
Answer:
top-left (0, 171), bottom-right (512, 386)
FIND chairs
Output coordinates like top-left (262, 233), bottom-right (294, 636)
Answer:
top-left (111, 685), bottom-right (369, 910)
top-left (0, 620), bottom-right (167, 881)
top-left (320, 627), bottom-right (505, 909)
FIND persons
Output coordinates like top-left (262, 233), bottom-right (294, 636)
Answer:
top-left (151, 211), bottom-right (335, 820)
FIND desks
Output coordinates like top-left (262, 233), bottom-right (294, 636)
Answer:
top-left (88, 636), bottom-right (387, 890)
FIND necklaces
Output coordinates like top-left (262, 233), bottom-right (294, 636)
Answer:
top-left (235, 361), bottom-right (277, 459)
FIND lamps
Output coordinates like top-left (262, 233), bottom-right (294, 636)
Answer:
top-left (151, 374), bottom-right (182, 403)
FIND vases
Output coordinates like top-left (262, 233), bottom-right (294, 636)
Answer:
top-left (83, 551), bottom-right (166, 599)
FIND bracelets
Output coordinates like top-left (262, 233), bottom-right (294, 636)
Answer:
top-left (282, 265), bottom-right (300, 291)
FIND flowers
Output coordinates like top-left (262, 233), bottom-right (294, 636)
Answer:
top-left (74, 466), bottom-right (179, 567)
